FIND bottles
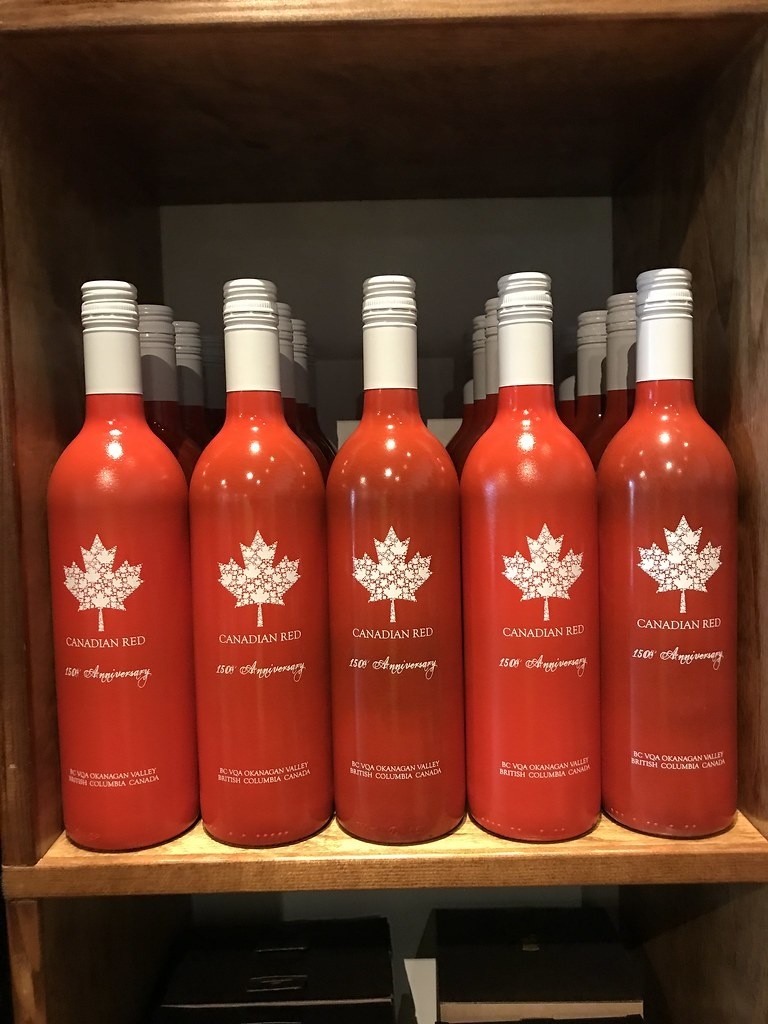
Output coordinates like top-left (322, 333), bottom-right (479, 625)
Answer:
top-left (327, 274), bottom-right (464, 845)
top-left (188, 279), bottom-right (338, 849)
top-left (445, 268), bottom-right (603, 844)
top-left (556, 269), bottom-right (738, 837)
top-left (46, 279), bottom-right (214, 854)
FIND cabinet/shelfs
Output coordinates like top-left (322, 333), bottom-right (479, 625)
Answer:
top-left (1, 1), bottom-right (768, 1023)
